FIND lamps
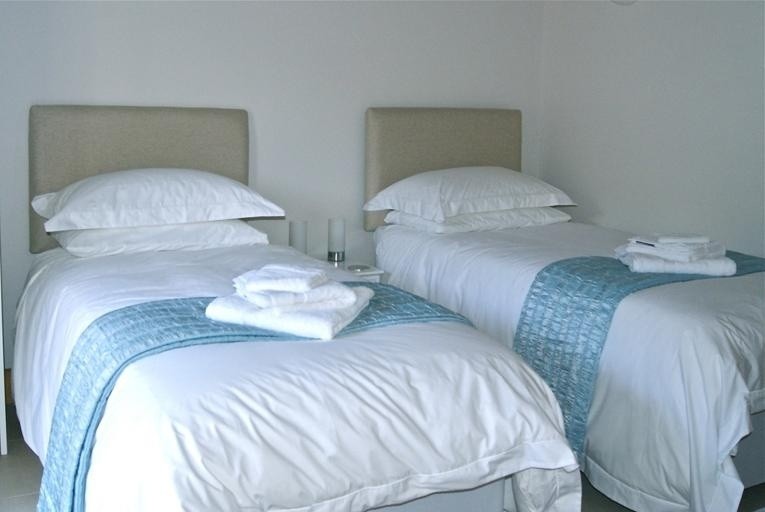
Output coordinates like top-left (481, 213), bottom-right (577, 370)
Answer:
top-left (288, 221), bottom-right (307, 253)
top-left (327, 217), bottom-right (346, 264)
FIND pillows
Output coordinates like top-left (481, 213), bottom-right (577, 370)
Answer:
top-left (31, 167), bottom-right (285, 234)
top-left (383, 206), bottom-right (573, 234)
top-left (48, 220), bottom-right (266, 258)
top-left (362, 163), bottom-right (579, 223)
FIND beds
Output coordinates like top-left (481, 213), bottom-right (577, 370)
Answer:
top-left (364, 106), bottom-right (764, 512)
top-left (10, 102), bottom-right (582, 512)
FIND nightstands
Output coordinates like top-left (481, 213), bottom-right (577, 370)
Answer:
top-left (342, 261), bottom-right (384, 283)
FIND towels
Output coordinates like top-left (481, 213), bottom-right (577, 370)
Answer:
top-left (232, 270), bottom-right (356, 312)
top-left (611, 248), bottom-right (737, 278)
top-left (655, 230), bottom-right (710, 244)
top-left (626, 240), bottom-right (726, 260)
top-left (203, 284), bottom-right (375, 342)
top-left (245, 263), bottom-right (326, 293)
